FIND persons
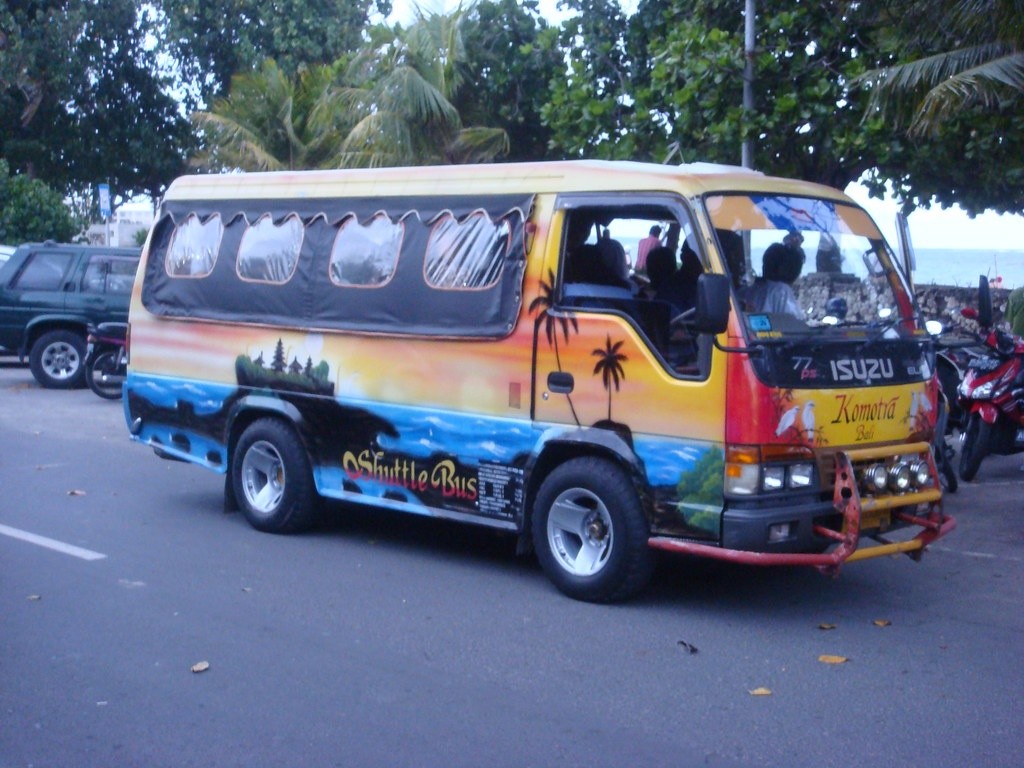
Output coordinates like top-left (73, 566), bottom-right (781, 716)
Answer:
top-left (747, 242), bottom-right (806, 323)
top-left (172, 215), bottom-right (843, 374)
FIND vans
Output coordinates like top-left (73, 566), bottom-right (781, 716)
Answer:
top-left (122, 161), bottom-right (959, 607)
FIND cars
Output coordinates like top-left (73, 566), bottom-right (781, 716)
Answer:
top-left (0, 244), bottom-right (125, 292)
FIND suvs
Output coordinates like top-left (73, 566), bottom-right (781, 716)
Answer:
top-left (0, 240), bottom-right (145, 391)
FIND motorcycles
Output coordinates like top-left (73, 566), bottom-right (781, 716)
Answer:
top-left (899, 279), bottom-right (1022, 483)
top-left (84, 321), bottom-right (127, 398)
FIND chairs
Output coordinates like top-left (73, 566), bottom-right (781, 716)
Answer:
top-left (567, 239), bottom-right (638, 295)
top-left (646, 247), bottom-right (677, 288)
